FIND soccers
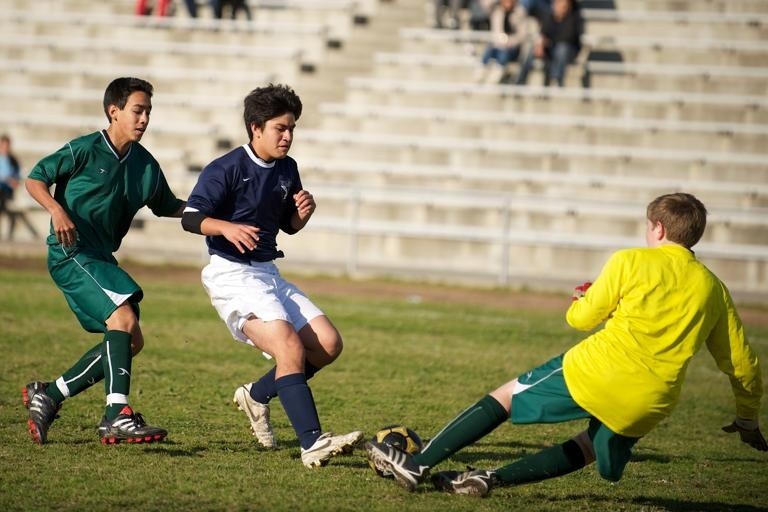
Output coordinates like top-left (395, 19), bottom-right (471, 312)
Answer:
top-left (367, 426), bottom-right (425, 480)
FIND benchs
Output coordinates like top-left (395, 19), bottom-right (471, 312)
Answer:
top-left (0, 1), bottom-right (768, 310)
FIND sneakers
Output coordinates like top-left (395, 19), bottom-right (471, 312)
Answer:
top-left (233, 382), bottom-right (276, 449)
top-left (99, 405), bottom-right (167, 443)
top-left (21, 381), bottom-right (60, 446)
top-left (301, 430), bottom-right (365, 468)
top-left (431, 467), bottom-right (498, 497)
top-left (366, 439), bottom-right (430, 491)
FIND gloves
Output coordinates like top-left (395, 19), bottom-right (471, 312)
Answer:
top-left (720, 420), bottom-right (767, 452)
top-left (571, 282), bottom-right (592, 302)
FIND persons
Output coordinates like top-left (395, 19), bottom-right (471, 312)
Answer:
top-left (20, 77), bottom-right (189, 446)
top-left (180, 82), bottom-right (366, 470)
top-left (185, 0), bottom-right (226, 19)
top-left (432, 1), bottom-right (615, 88)
top-left (366, 191), bottom-right (767, 498)
top-left (229, 1), bottom-right (254, 22)
top-left (0, 134), bottom-right (21, 212)
top-left (136, 0), bottom-right (172, 18)
